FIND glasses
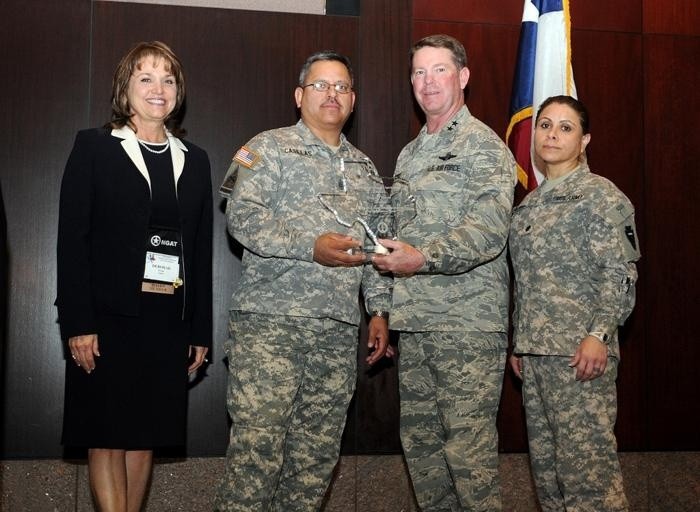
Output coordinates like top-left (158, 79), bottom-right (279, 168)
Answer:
top-left (302, 80), bottom-right (352, 94)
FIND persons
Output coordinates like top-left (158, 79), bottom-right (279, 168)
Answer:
top-left (371, 34), bottom-right (516, 512)
top-left (55, 40), bottom-right (213, 512)
top-left (509, 95), bottom-right (641, 512)
top-left (210, 51), bottom-right (392, 512)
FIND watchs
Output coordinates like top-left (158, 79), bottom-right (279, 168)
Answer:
top-left (589, 330), bottom-right (609, 344)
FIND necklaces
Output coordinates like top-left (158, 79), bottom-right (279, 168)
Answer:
top-left (136, 136), bottom-right (172, 156)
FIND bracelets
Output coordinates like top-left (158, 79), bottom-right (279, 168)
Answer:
top-left (374, 310), bottom-right (388, 318)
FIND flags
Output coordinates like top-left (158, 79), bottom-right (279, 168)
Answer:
top-left (504, 0), bottom-right (579, 196)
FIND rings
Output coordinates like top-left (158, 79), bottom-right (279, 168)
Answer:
top-left (203, 357), bottom-right (208, 363)
top-left (73, 354), bottom-right (77, 361)
